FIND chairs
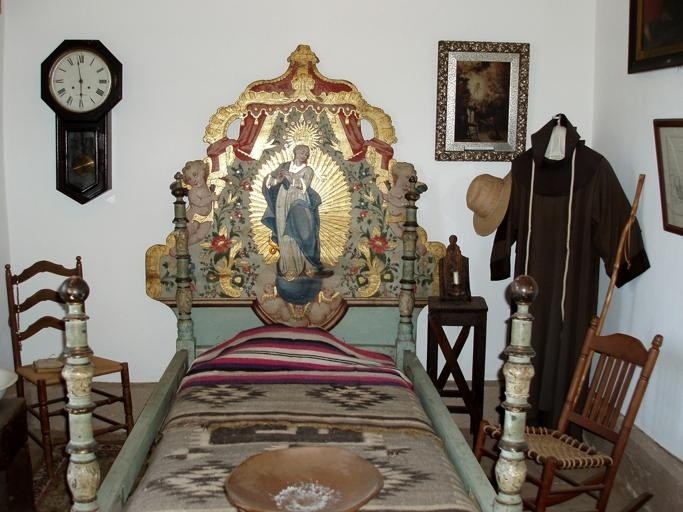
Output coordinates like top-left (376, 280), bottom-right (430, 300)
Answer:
top-left (474, 316), bottom-right (663, 512)
top-left (5, 256), bottom-right (134, 477)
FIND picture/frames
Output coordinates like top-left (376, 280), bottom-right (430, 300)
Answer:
top-left (652, 119), bottom-right (683, 236)
top-left (628, 1), bottom-right (683, 73)
top-left (434, 39), bottom-right (530, 162)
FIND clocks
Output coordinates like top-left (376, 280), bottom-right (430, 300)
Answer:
top-left (40, 40), bottom-right (122, 204)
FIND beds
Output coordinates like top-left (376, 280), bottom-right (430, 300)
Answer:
top-left (60, 46), bottom-right (539, 512)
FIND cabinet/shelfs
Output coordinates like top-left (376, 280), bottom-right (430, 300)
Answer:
top-left (0, 395), bottom-right (36, 511)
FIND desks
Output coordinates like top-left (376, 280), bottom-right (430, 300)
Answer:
top-left (427, 295), bottom-right (488, 435)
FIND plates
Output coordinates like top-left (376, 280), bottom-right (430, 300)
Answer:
top-left (222, 444), bottom-right (385, 512)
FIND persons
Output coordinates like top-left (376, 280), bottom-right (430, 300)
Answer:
top-left (169, 160), bottom-right (219, 257)
top-left (384, 163), bottom-right (427, 255)
top-left (309, 284), bottom-right (342, 325)
top-left (256, 283), bottom-right (289, 321)
top-left (261, 145), bottom-right (324, 280)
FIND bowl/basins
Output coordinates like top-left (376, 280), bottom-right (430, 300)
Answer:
top-left (0, 366), bottom-right (19, 403)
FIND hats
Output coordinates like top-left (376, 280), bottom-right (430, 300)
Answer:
top-left (466, 170), bottom-right (513, 237)
